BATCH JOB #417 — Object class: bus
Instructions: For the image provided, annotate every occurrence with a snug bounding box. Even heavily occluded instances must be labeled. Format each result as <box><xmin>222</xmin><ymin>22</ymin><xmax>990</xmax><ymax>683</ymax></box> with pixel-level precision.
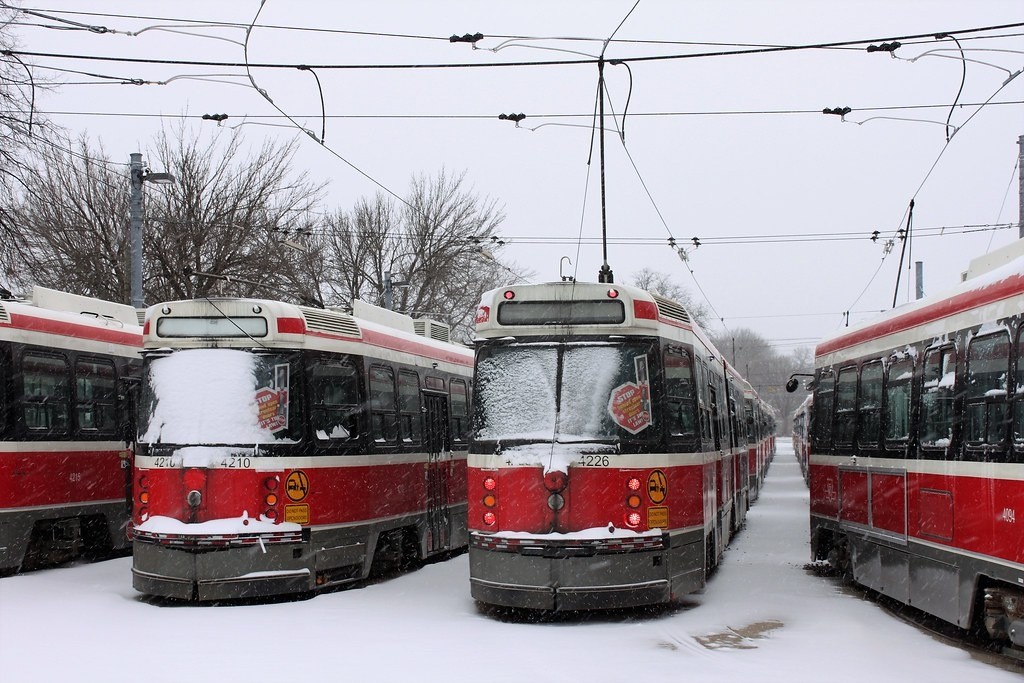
<box><xmin>128</xmin><ymin>297</ymin><xmax>472</xmax><ymax>601</ymax></box>
<box><xmin>0</xmin><ymin>278</ymin><xmax>167</xmax><ymax>575</ymax></box>
<box><xmin>789</xmin><ymin>238</ymin><xmax>1024</xmax><ymax>665</ymax></box>
<box><xmin>464</xmin><ymin>272</ymin><xmax>782</xmax><ymax>628</ymax></box>
